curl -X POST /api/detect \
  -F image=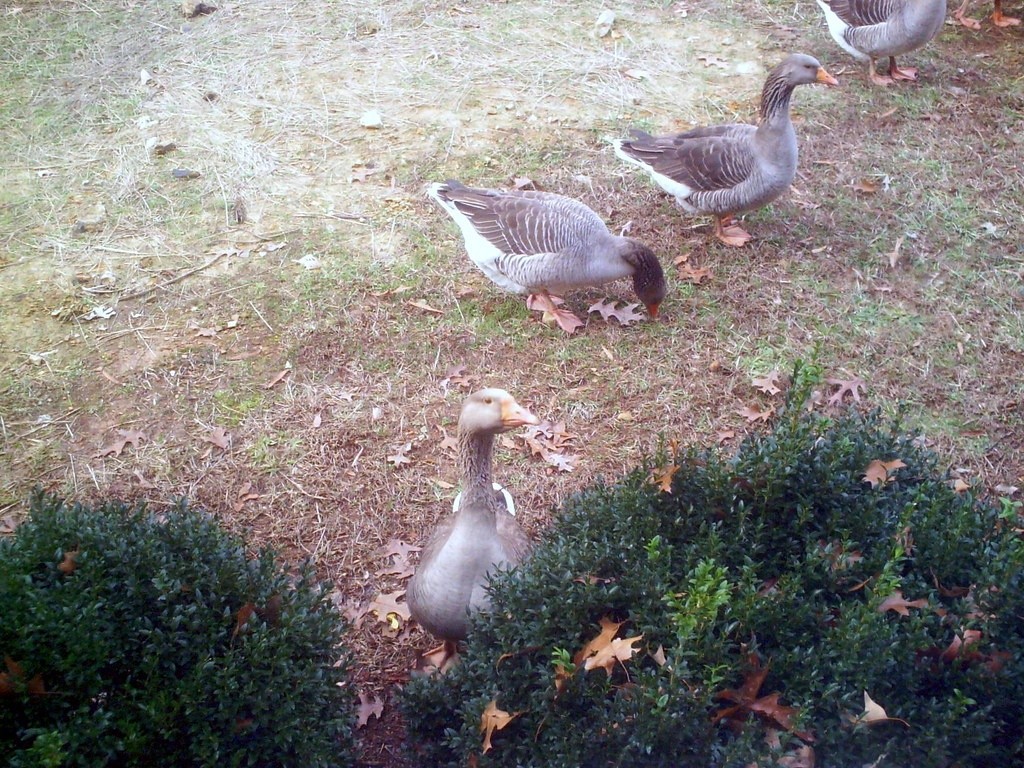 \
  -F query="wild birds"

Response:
[405,388,542,677]
[816,0,1020,87]
[428,177,665,337]
[613,53,839,247]
